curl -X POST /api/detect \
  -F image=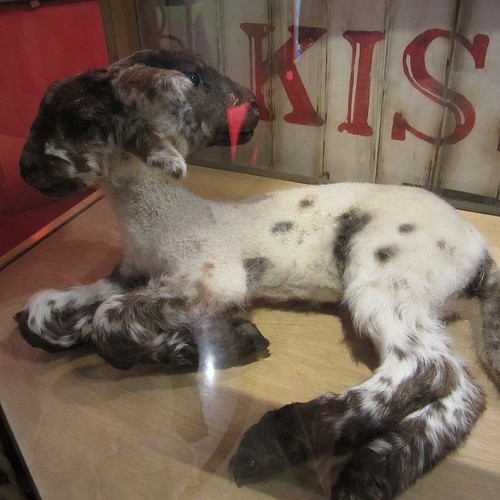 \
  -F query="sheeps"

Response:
[9,49,499,497]
